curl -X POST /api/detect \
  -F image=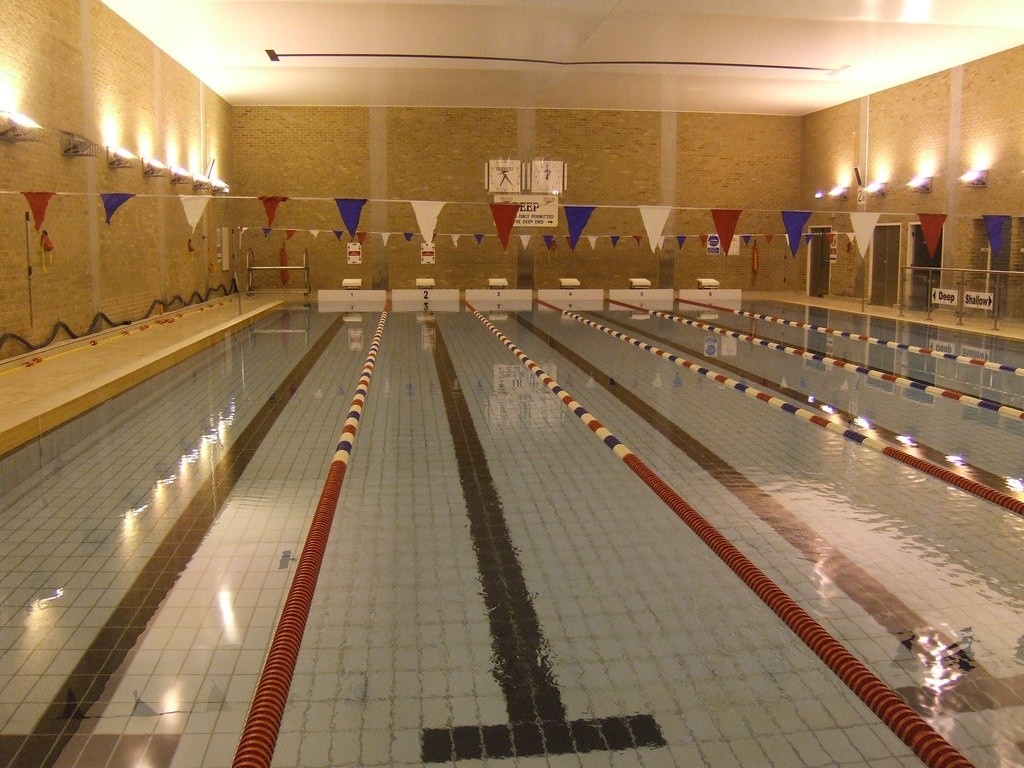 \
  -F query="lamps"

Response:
[211,180,230,195]
[107,147,138,168]
[170,166,192,185]
[906,177,932,193]
[142,158,167,177]
[192,173,209,190]
[864,182,887,198]
[827,187,849,200]
[59,130,100,158]
[0,110,43,142]
[957,170,988,188]
